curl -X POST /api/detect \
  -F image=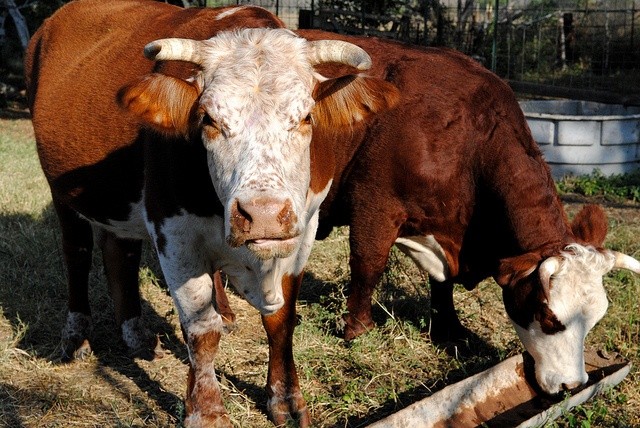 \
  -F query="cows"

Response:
[209,28,640,395]
[22,1,371,427]
